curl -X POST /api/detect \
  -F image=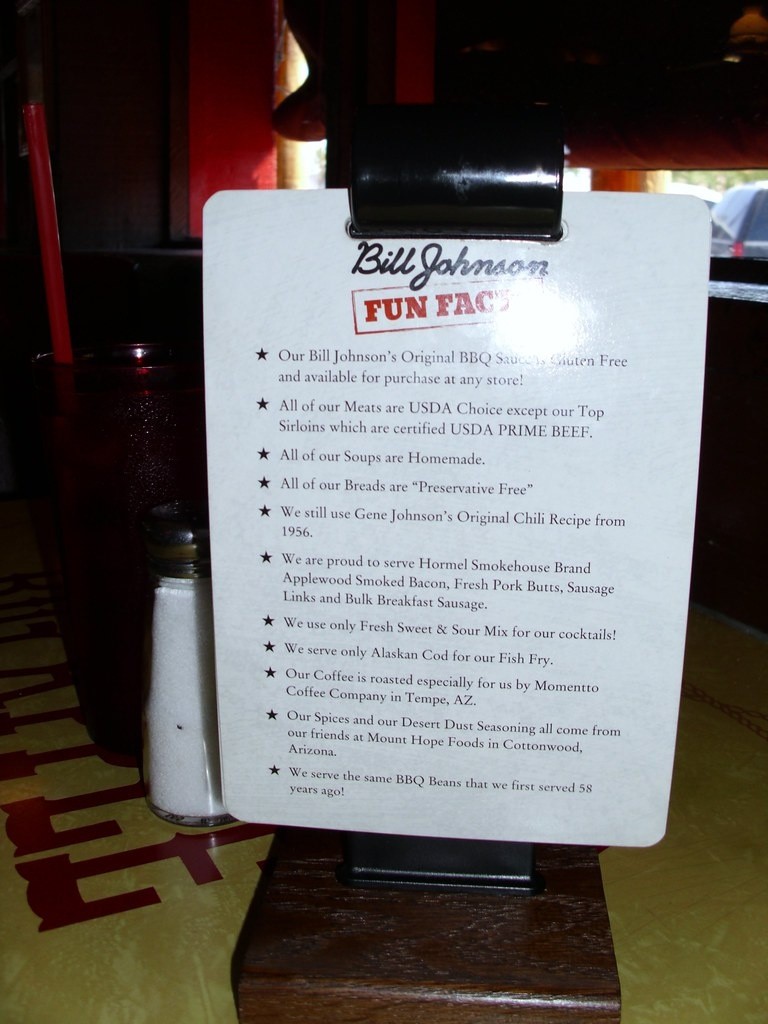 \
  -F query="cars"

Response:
[707,181,768,262]
